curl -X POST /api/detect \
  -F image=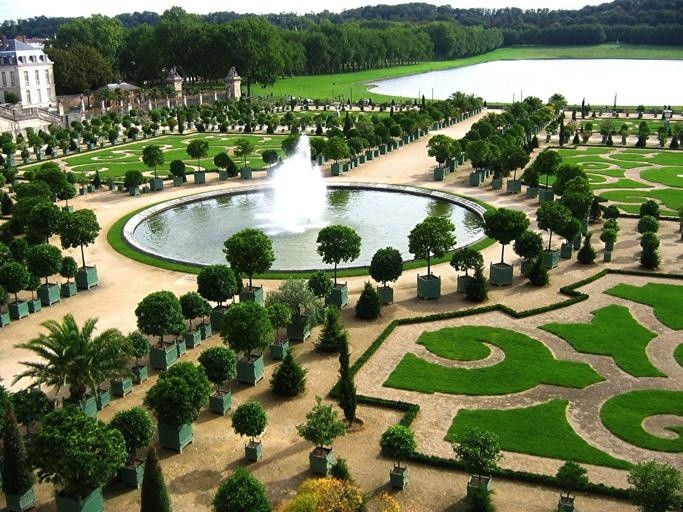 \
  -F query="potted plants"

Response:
[310,98,434,176]
[197,227,335,417]
[636,104,645,117]
[450,246,488,301]
[230,402,269,463]
[280,98,312,133]
[315,224,362,312]
[185,139,209,184]
[407,216,457,300]
[377,425,417,489]
[234,138,255,179]
[261,148,278,177]
[426,91,483,131]
[425,93,568,186]
[213,152,233,181]
[294,396,346,477]
[556,460,589,512]
[142,145,164,192]
[1,111,123,168]
[451,427,504,501]
[612,105,617,114]
[107,170,145,196]
[78,169,102,196]
[122,101,279,143]
[1,291,213,512]
[168,159,187,186]
[479,147,594,287]
[1,198,102,327]
[370,247,403,304]
